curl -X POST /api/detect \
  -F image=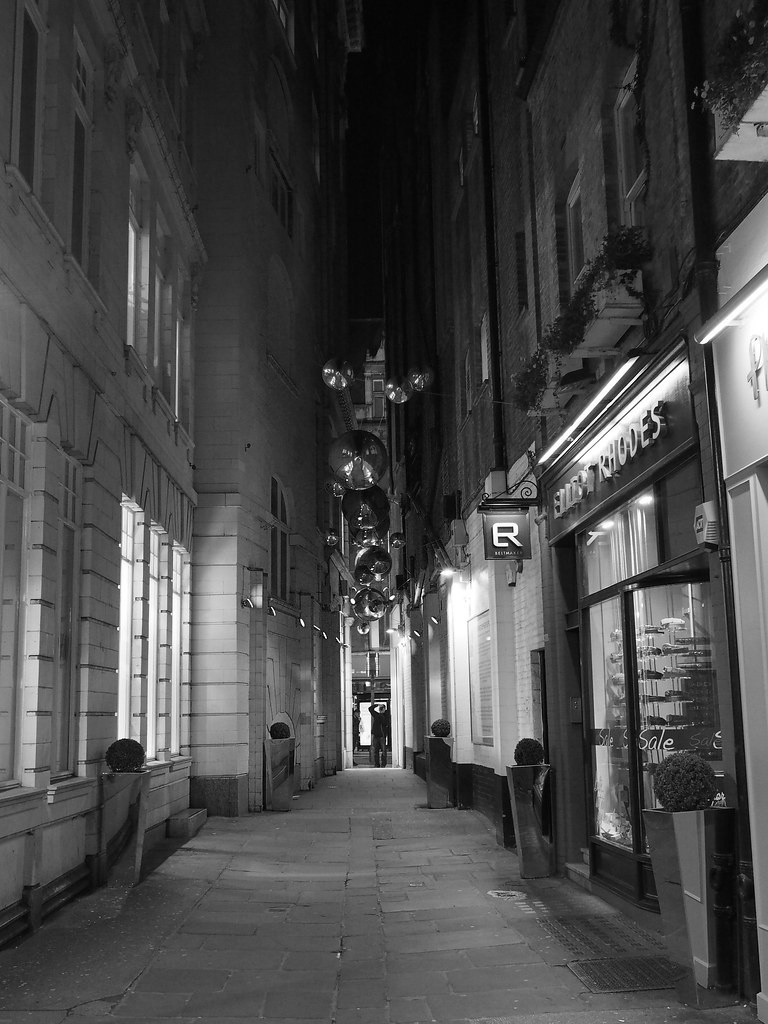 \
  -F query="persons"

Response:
[368,704,391,768]
[353,709,361,766]
